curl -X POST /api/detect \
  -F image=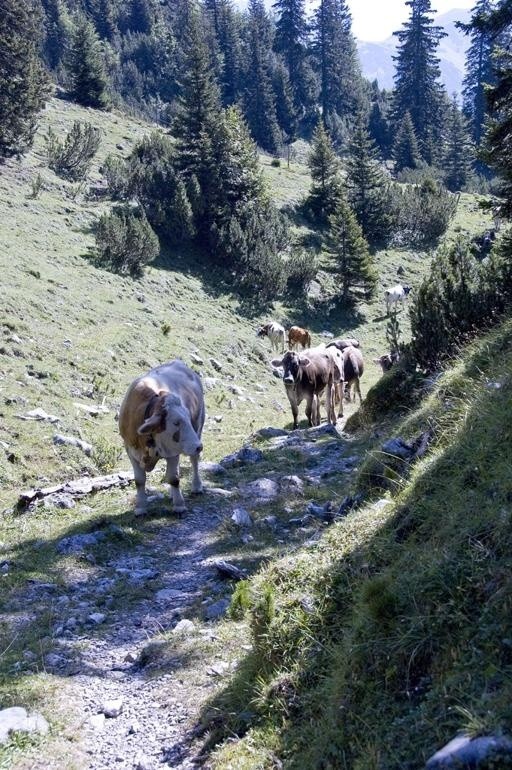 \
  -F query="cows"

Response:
[119,360,205,517]
[288,326,311,351]
[255,321,285,354]
[373,350,403,374]
[384,284,412,316]
[271,339,364,429]
